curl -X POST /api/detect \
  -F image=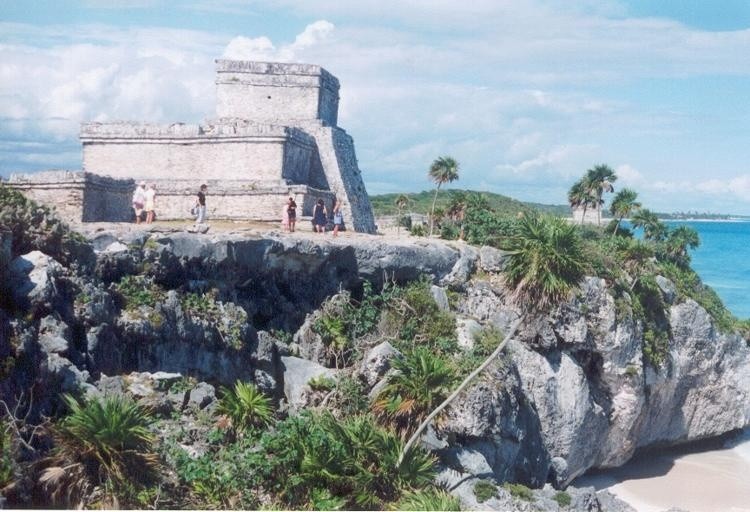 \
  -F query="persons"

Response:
[195,182,207,223]
[144,184,157,224]
[311,198,328,236]
[287,197,297,232]
[332,198,343,238]
[132,180,145,224]
